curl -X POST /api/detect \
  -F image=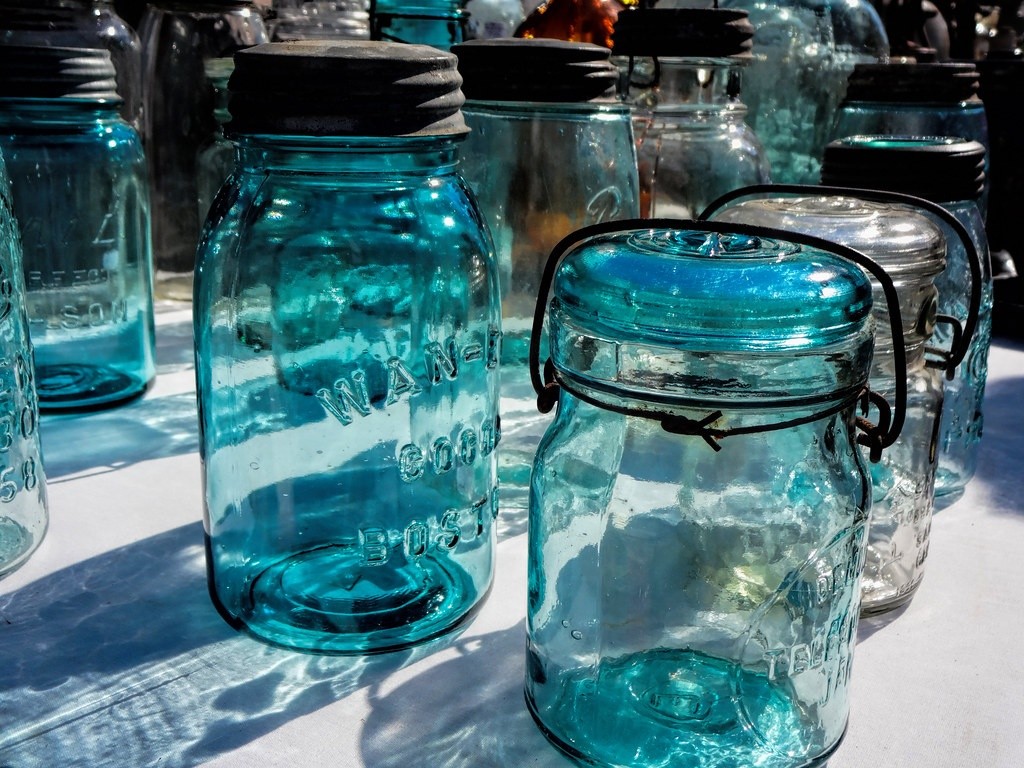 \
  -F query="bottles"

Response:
[0,0,993,768]
